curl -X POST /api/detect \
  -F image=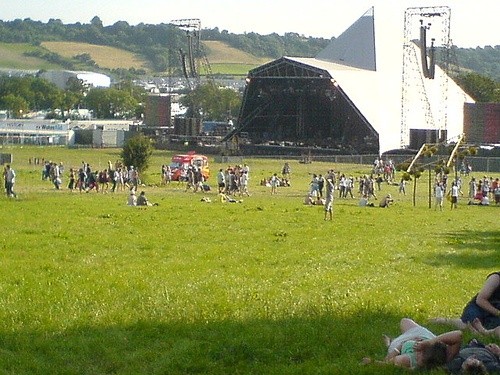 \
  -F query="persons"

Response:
[1,156,500,211]
[428,271,500,330]
[465,318,500,338]
[445,339,500,375]
[324,178,334,221]
[357,317,463,370]
